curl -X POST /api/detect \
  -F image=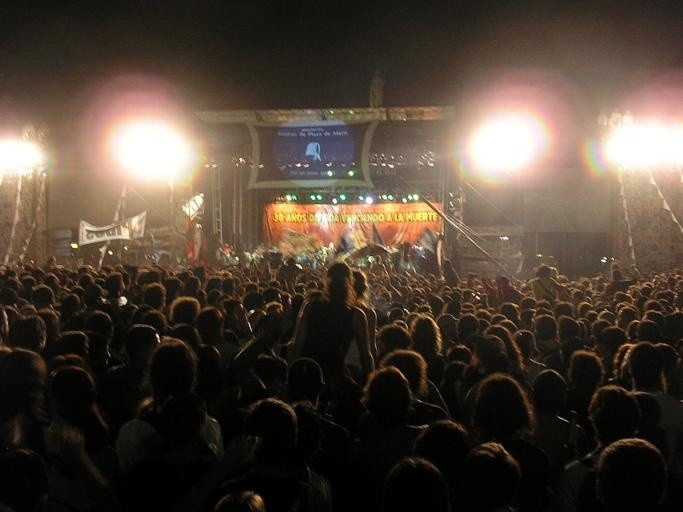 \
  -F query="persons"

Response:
[1,242,683,511]
